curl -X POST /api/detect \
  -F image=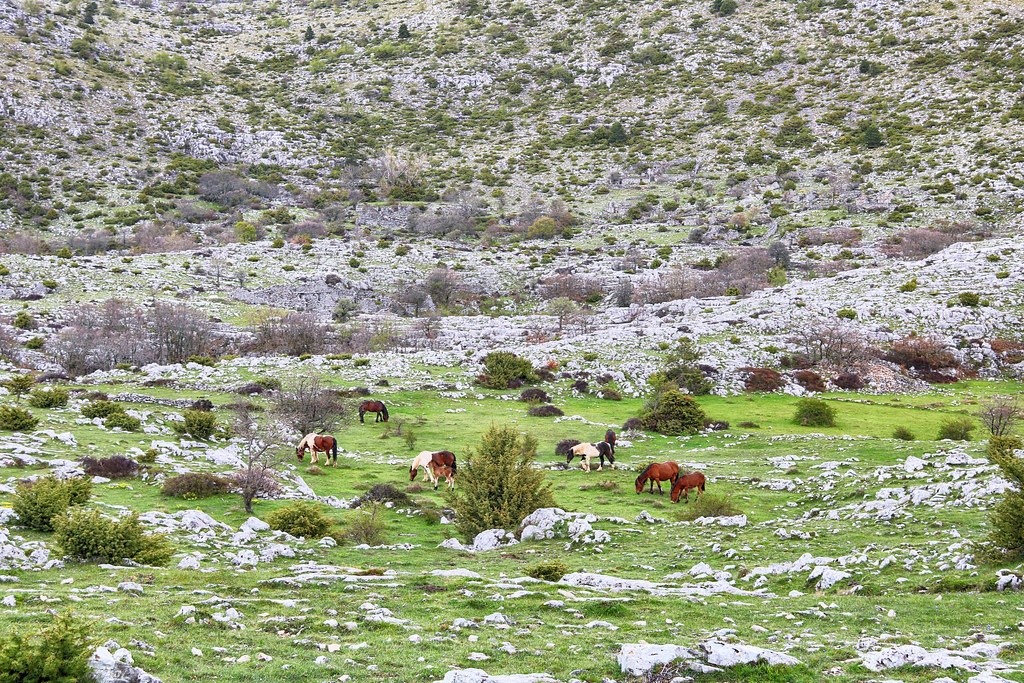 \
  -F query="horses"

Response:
[409,451,457,491]
[567,429,616,474]
[296,433,337,467]
[359,401,389,425]
[635,462,706,503]
